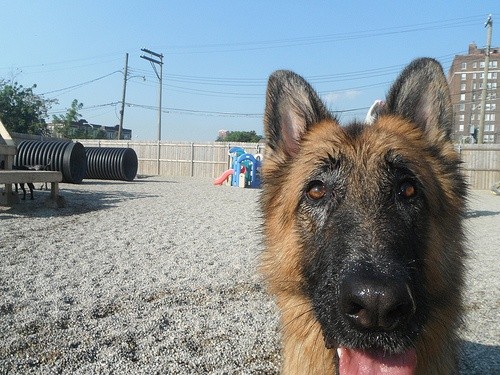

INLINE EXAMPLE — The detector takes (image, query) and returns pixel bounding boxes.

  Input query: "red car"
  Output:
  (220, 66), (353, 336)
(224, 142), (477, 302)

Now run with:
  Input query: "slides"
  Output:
(212, 168), (233, 185)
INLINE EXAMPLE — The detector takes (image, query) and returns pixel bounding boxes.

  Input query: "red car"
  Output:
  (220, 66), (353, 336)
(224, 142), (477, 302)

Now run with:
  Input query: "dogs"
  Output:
(257, 56), (467, 375)
